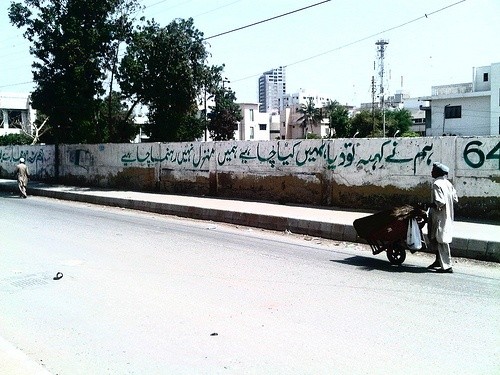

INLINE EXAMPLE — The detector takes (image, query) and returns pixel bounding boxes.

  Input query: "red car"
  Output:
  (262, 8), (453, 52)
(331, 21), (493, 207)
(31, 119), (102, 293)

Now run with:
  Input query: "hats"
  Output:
(19, 158), (25, 162)
(433, 161), (450, 173)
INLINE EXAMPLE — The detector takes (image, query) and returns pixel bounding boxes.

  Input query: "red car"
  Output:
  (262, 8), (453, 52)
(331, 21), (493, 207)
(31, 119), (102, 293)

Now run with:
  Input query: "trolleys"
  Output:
(354, 199), (429, 264)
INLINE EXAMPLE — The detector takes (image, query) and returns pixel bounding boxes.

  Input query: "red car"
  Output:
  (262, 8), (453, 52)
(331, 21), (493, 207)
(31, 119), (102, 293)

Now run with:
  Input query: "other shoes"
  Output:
(427, 261), (441, 269)
(436, 267), (453, 273)
(19, 195), (27, 198)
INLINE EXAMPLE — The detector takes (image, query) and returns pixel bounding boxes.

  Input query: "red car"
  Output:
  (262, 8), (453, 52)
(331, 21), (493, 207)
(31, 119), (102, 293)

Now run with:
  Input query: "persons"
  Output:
(421, 162), (458, 273)
(14, 158), (28, 199)
